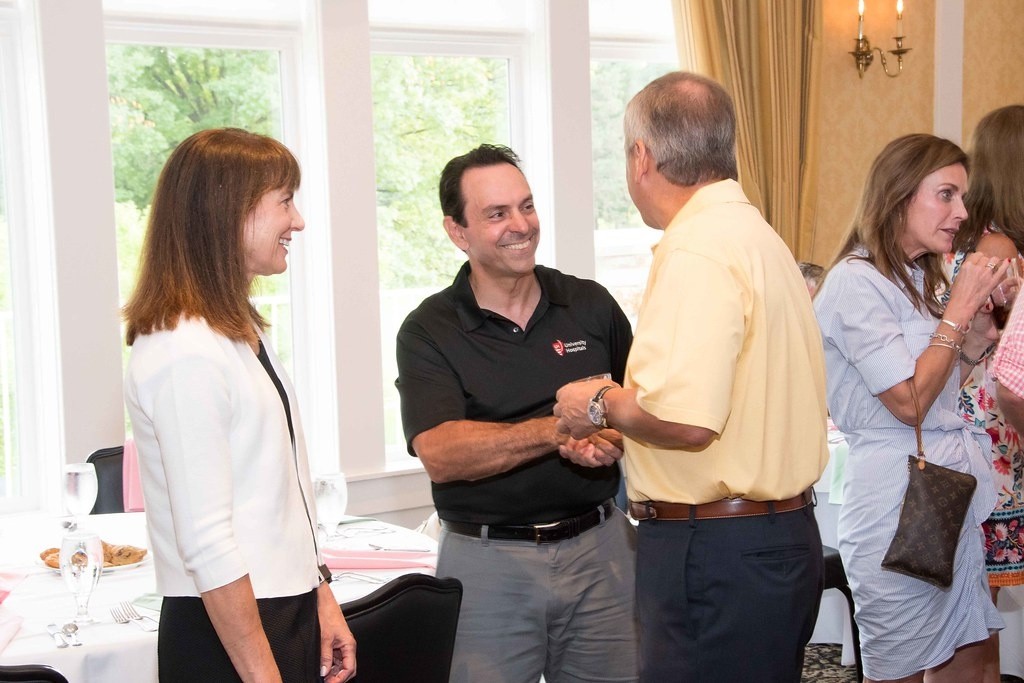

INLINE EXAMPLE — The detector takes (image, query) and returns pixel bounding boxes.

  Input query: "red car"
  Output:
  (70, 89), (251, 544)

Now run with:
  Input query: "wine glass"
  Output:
(61, 533), (104, 626)
(311, 471), (351, 558)
(60, 462), (99, 541)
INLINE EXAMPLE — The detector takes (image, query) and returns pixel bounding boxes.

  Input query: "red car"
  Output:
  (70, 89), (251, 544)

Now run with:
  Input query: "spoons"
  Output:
(64, 620), (83, 645)
(367, 543), (429, 551)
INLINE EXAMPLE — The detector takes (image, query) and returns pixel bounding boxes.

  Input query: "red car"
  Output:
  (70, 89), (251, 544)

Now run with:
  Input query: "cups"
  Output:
(989, 256), (1020, 308)
(567, 372), (613, 382)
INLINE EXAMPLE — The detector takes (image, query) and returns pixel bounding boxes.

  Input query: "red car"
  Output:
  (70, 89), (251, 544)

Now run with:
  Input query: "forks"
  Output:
(109, 599), (160, 633)
(336, 526), (396, 538)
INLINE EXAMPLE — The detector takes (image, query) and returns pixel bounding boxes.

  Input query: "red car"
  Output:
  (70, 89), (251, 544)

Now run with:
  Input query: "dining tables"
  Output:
(0, 511), (439, 683)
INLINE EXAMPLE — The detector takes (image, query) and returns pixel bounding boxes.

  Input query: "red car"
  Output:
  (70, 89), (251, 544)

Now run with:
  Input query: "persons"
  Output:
(396, 145), (638, 682)
(799, 104), (1024, 682)
(552, 71), (831, 683)
(125, 127), (358, 683)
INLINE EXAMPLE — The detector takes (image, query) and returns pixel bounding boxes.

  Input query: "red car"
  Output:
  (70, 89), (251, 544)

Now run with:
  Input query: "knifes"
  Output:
(46, 624), (67, 649)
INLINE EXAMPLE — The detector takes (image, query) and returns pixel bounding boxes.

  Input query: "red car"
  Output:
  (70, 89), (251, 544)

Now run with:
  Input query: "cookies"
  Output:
(39, 539), (147, 570)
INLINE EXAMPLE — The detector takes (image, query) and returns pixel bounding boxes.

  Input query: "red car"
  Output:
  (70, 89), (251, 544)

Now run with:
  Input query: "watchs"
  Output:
(588, 384), (614, 428)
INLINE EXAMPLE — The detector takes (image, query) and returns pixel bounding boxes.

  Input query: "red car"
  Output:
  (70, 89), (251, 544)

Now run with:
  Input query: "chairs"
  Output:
(339, 573), (464, 683)
(821, 543), (863, 683)
(85, 446), (124, 515)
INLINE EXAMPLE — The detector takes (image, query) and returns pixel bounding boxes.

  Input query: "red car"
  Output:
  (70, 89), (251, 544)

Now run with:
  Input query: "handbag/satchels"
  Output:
(880, 374), (977, 590)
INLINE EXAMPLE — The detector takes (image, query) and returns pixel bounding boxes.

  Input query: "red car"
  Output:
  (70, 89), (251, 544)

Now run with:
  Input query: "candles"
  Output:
(856, 0), (865, 39)
(893, 0), (905, 37)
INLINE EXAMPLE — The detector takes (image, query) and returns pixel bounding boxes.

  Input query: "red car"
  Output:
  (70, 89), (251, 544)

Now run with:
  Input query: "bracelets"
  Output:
(927, 316), (971, 361)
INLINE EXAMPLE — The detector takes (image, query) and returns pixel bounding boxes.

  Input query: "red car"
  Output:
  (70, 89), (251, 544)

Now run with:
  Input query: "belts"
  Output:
(629, 486), (814, 521)
(438, 497), (616, 547)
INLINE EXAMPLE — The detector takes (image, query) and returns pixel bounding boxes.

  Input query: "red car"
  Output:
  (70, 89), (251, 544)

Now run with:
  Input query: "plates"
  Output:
(35, 543), (153, 573)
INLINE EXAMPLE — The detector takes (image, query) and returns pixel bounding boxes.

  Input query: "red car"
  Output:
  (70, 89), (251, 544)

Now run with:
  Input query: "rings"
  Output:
(985, 263), (998, 272)
